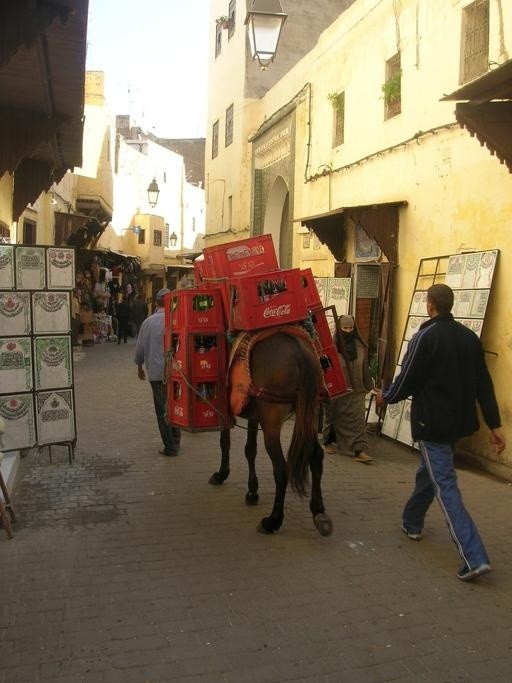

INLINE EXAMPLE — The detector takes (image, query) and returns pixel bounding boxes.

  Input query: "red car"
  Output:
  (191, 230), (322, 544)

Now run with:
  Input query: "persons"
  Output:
(322, 314), (376, 462)
(115, 296), (130, 344)
(134, 289), (181, 456)
(132, 294), (148, 335)
(374, 284), (506, 581)
(72, 289), (82, 346)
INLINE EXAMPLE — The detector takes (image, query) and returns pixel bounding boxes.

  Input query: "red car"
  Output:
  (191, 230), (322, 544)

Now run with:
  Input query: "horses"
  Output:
(208, 331), (334, 537)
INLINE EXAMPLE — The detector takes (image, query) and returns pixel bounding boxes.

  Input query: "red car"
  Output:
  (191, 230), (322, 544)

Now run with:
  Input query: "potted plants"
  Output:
(216, 16), (230, 29)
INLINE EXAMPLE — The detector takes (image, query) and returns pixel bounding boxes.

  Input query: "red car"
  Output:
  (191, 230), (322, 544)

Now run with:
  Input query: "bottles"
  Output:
(197, 383), (214, 400)
(194, 334), (217, 352)
(321, 357), (332, 370)
(301, 277), (308, 288)
(193, 295), (214, 311)
(257, 279), (287, 303)
(172, 335), (180, 353)
(173, 381), (181, 400)
(171, 296), (177, 312)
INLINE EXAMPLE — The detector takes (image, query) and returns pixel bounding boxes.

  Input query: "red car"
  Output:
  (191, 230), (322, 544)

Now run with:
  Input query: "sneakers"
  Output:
(456, 564), (491, 581)
(401, 526), (423, 539)
(325, 446), (335, 454)
(355, 452), (375, 463)
(160, 447), (174, 455)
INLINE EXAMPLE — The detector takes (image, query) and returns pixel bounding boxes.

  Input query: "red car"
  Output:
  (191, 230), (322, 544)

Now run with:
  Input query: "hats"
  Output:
(157, 288), (171, 300)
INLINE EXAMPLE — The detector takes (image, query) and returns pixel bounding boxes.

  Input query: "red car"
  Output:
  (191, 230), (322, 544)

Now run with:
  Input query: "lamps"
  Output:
(244, 0), (288, 71)
(170, 232), (177, 246)
(147, 177), (160, 208)
